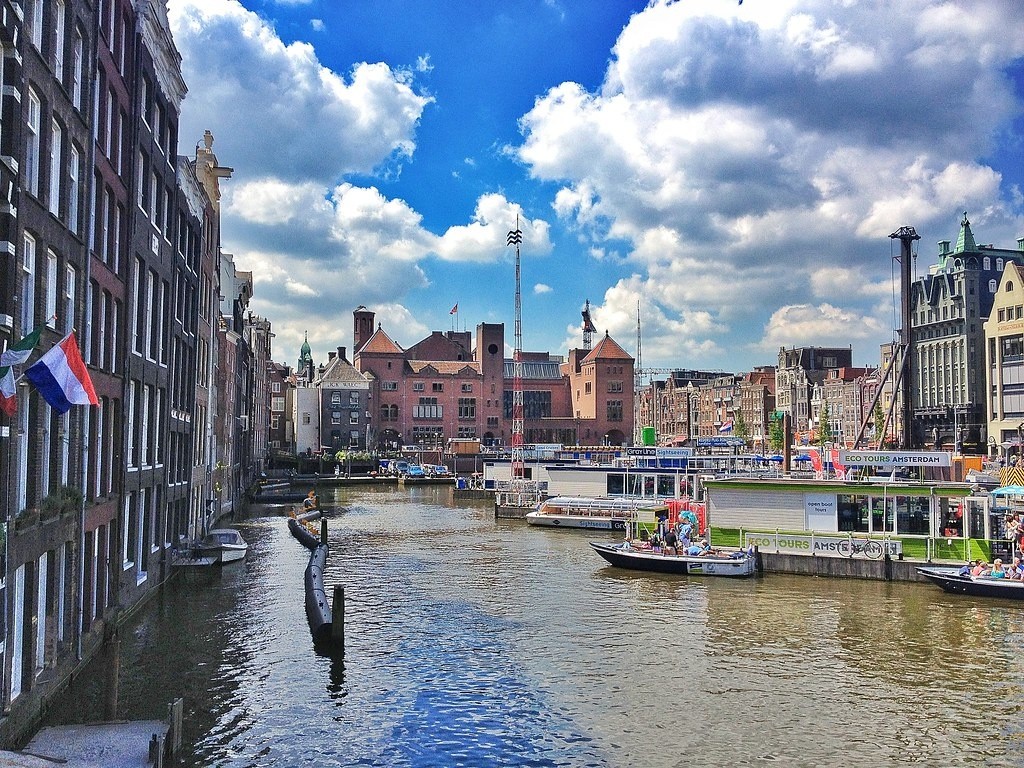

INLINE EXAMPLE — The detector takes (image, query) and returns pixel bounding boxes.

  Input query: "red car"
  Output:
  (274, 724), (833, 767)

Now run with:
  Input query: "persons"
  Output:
(359, 435), (1024, 578)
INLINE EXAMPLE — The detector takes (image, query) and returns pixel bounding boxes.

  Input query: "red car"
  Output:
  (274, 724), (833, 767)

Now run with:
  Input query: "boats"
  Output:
(525, 495), (660, 529)
(915, 566), (1024, 599)
(587, 538), (755, 577)
(194, 528), (247, 564)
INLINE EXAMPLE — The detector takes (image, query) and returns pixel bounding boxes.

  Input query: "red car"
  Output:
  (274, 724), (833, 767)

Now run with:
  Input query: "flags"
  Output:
(0, 320), (46, 421)
(718, 419), (732, 431)
(22, 331), (103, 418)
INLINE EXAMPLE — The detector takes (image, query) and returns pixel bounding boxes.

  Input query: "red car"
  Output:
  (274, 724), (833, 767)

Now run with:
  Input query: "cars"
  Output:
(370, 457), (455, 480)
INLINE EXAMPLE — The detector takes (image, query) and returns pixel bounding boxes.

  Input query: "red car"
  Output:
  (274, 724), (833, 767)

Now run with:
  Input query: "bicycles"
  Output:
(836, 529), (883, 559)
